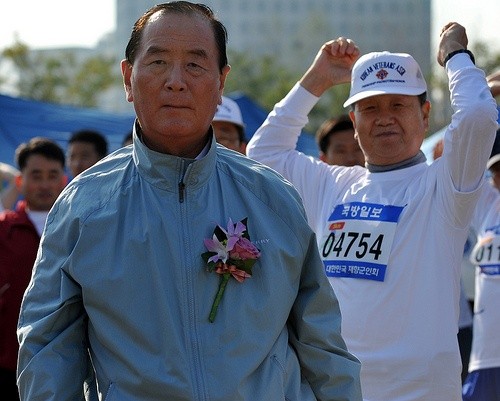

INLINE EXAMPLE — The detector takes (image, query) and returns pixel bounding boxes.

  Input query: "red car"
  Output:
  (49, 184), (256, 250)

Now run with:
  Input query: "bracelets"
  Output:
(443, 49), (475, 65)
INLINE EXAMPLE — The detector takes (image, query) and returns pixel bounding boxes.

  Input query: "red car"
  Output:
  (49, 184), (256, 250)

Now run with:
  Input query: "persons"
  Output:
(244, 22), (497, 401)
(0, 71), (499, 401)
(16, 2), (364, 401)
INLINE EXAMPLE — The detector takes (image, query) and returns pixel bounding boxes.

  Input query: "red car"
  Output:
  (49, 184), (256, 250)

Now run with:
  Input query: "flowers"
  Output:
(200, 217), (262, 324)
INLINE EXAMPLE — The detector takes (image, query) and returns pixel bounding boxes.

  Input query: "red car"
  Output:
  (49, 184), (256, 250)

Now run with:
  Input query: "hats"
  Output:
(343, 51), (428, 108)
(212, 94), (248, 132)
